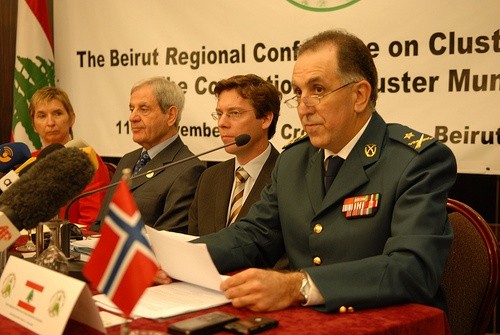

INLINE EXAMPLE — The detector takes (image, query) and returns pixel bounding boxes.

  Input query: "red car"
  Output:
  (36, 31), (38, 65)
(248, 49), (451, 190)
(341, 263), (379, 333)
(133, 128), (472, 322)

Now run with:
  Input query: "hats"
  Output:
(0, 142), (31, 174)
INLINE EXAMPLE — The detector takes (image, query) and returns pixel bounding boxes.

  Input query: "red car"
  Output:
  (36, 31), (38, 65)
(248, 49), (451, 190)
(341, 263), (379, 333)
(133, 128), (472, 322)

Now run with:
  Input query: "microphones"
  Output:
(0, 141), (93, 254)
(60, 134), (251, 258)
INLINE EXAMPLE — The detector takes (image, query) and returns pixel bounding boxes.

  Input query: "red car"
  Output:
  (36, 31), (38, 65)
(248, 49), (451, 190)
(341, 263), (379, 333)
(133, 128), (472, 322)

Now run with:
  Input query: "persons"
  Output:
(89, 76), (206, 241)
(187, 72), (284, 240)
(20, 86), (113, 237)
(149, 29), (458, 335)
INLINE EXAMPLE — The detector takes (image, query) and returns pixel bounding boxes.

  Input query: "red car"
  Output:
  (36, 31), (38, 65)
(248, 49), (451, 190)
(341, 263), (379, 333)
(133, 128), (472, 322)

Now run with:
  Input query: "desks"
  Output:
(0, 229), (451, 335)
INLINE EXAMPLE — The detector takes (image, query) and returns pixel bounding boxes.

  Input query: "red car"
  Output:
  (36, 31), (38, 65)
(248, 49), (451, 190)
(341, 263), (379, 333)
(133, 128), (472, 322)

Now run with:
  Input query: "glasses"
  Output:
(211, 109), (262, 121)
(285, 80), (361, 109)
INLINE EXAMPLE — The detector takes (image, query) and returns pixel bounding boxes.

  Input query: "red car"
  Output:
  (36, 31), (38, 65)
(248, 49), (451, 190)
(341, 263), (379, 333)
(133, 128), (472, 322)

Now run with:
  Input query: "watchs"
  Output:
(300, 271), (312, 302)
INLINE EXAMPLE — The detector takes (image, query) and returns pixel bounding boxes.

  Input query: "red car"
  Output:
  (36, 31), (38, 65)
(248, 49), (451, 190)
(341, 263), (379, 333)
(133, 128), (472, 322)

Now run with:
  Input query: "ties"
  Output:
(131, 150), (150, 173)
(323, 157), (344, 199)
(226, 168), (250, 229)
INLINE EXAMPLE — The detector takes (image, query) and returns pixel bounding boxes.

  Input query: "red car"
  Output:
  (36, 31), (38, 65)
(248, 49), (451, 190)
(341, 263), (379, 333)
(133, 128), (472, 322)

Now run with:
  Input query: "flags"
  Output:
(83, 181), (162, 319)
(8, 0), (56, 160)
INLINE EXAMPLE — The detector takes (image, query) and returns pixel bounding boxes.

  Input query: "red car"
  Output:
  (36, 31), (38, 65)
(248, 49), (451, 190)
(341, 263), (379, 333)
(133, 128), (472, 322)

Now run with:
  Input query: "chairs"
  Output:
(440, 198), (500, 335)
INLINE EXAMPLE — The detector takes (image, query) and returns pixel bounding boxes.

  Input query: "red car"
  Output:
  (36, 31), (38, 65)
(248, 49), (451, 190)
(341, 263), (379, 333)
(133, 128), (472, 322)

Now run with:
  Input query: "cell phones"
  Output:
(167, 311), (238, 335)
(224, 316), (280, 335)
